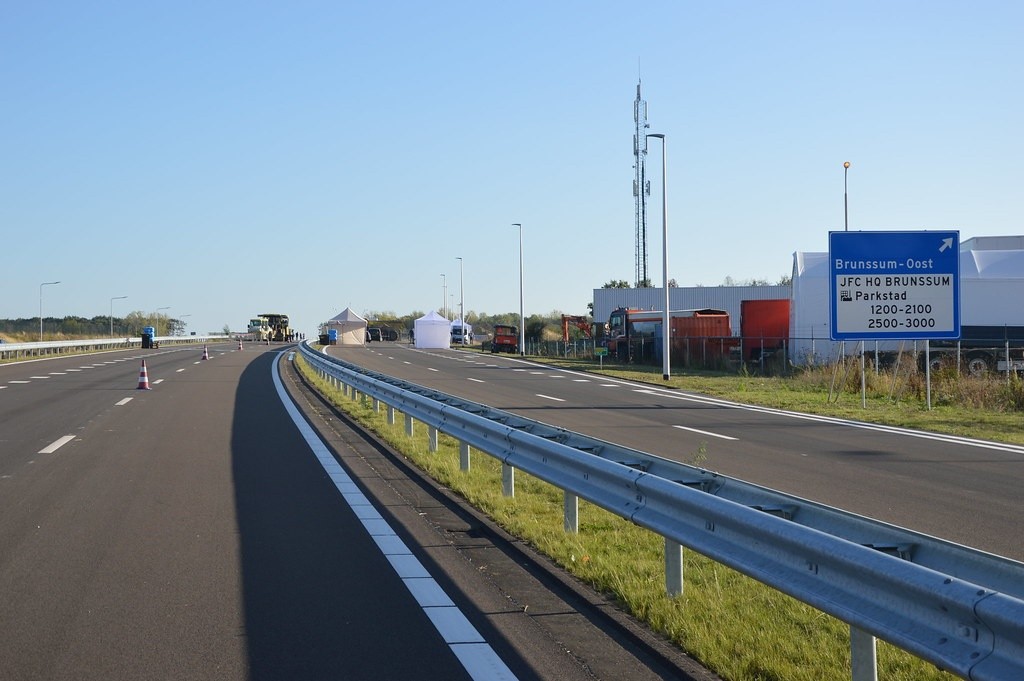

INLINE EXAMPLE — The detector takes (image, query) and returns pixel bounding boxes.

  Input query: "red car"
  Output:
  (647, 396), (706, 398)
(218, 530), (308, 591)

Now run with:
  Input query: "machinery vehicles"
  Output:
(558, 314), (611, 357)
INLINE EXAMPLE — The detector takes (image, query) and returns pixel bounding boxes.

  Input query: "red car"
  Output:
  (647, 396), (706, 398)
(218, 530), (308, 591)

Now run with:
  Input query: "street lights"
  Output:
(455, 256), (464, 348)
(110, 296), (128, 338)
(843, 161), (850, 230)
(156, 307), (170, 337)
(177, 314), (191, 335)
(646, 134), (671, 381)
(439, 273), (454, 323)
(511, 223), (525, 356)
(39, 281), (60, 341)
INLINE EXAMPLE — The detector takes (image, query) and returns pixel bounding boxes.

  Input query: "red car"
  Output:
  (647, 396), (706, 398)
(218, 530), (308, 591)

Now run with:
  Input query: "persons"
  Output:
(408, 330), (415, 344)
(300, 333), (306, 341)
(470, 331), (475, 345)
(295, 332), (299, 341)
(292, 329), (295, 341)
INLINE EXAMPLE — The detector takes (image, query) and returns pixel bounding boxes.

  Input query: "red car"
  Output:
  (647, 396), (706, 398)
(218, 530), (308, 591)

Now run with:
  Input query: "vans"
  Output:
(368, 328), (382, 342)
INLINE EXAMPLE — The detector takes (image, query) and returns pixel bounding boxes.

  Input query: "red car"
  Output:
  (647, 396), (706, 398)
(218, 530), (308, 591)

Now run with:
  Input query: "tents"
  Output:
(788, 250), (924, 366)
(328, 307), (368, 345)
(449, 318), (472, 335)
(413, 310), (451, 349)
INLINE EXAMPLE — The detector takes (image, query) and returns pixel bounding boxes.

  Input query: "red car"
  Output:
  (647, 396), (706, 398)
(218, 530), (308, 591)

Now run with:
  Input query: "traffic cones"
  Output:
(200, 344), (208, 360)
(238, 339), (243, 351)
(136, 360), (151, 389)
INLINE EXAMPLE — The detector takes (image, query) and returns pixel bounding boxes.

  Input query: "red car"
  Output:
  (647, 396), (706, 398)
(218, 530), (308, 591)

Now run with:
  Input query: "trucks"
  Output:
(248, 313), (293, 342)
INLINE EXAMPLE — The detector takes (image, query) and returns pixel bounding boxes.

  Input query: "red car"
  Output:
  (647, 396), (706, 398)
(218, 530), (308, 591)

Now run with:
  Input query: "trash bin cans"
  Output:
(318, 334), (329, 345)
(140, 333), (150, 349)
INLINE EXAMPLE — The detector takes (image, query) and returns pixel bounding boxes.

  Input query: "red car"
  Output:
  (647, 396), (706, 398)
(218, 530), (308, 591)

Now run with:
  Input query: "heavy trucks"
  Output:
(606, 306), (731, 361)
(450, 323), (471, 344)
(481, 324), (518, 353)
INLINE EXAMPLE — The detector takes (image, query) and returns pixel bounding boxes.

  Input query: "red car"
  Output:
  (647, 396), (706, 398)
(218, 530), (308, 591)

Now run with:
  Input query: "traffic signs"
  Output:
(828, 230), (960, 342)
